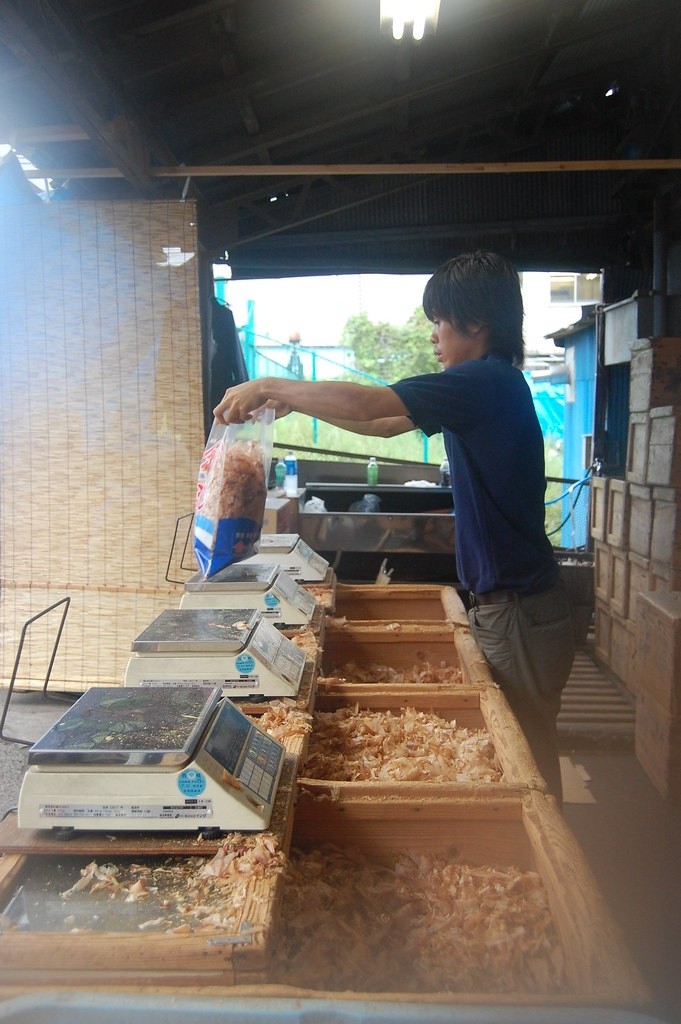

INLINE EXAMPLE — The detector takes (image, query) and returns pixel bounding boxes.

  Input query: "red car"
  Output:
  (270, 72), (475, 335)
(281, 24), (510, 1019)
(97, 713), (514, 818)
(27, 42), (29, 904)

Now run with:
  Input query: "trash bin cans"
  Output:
(555, 552), (596, 646)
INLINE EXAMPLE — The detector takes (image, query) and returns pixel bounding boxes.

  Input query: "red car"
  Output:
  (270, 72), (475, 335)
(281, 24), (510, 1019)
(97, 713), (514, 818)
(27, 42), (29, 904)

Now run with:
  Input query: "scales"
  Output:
(237, 532), (330, 584)
(179, 563), (318, 630)
(125, 609), (307, 704)
(18, 686), (287, 840)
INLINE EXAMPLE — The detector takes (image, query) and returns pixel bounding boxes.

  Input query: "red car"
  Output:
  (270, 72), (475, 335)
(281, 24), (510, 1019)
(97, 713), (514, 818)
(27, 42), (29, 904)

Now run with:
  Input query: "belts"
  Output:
(469, 571), (558, 608)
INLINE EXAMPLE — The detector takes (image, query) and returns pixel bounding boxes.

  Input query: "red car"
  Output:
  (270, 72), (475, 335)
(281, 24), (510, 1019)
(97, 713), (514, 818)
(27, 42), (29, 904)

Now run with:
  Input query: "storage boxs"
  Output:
(588, 335), (681, 805)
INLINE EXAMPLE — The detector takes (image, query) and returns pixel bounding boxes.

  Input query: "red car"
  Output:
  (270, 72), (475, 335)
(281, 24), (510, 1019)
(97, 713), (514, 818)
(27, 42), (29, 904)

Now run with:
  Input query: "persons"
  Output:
(213, 249), (577, 813)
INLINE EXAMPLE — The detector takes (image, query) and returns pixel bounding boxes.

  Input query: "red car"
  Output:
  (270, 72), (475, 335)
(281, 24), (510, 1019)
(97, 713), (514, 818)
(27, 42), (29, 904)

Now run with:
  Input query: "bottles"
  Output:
(367, 457), (378, 486)
(438, 458), (452, 488)
(274, 457), (287, 496)
(285, 450), (298, 498)
